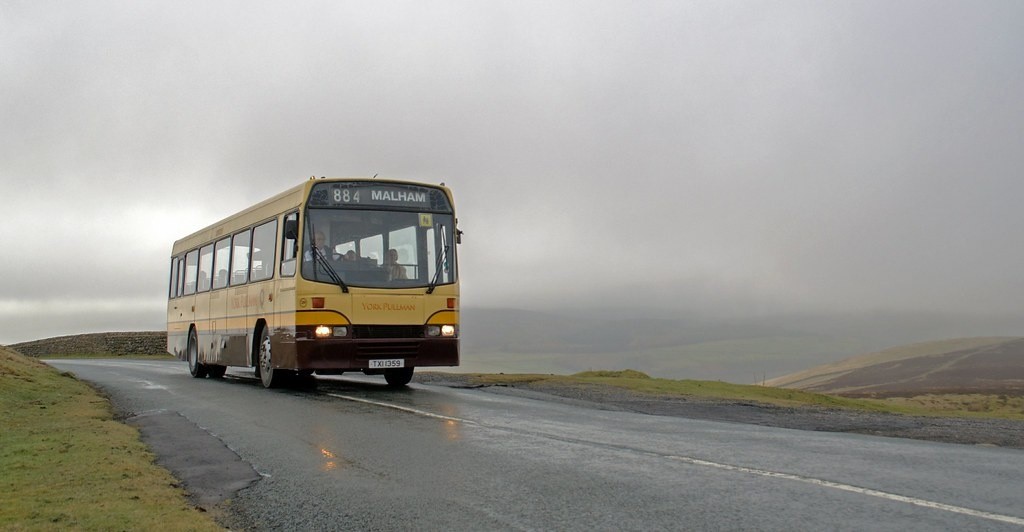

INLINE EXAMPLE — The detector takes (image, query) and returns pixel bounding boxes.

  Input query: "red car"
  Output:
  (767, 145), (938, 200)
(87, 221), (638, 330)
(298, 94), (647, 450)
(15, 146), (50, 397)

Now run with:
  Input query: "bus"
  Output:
(167, 174), (460, 388)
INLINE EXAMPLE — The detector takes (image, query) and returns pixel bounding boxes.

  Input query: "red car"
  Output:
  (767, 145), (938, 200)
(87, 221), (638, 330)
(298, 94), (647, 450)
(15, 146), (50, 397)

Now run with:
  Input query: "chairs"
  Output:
(186, 265), (263, 294)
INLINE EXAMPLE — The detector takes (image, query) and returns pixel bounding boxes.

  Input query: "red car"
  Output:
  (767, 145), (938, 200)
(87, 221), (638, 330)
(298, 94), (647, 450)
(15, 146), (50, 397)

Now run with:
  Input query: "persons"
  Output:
(342, 250), (357, 261)
(380, 249), (408, 280)
(200, 271), (209, 290)
(303, 232), (334, 261)
(219, 269), (228, 287)
